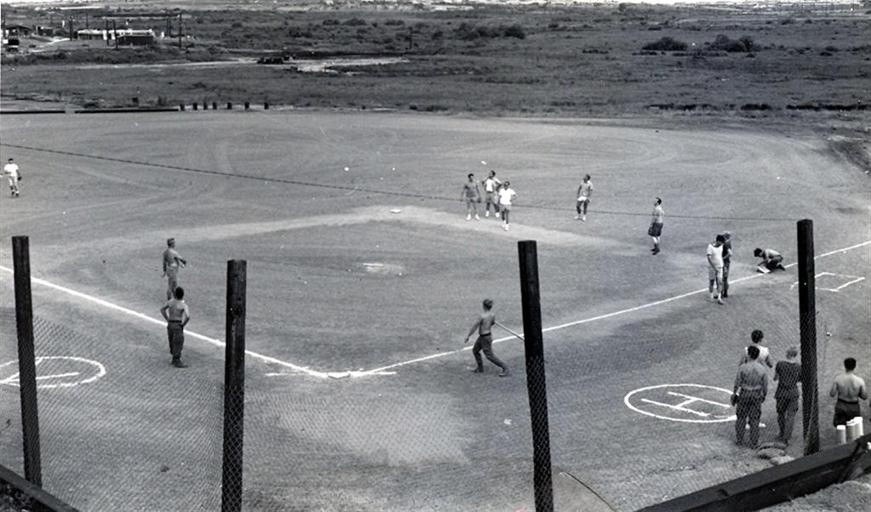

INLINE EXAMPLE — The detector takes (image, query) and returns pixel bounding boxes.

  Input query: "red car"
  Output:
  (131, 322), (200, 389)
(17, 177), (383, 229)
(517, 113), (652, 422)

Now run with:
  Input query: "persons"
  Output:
(161, 287), (190, 367)
(574, 174), (593, 221)
(464, 299), (509, 377)
(647, 196), (665, 254)
(461, 170), (518, 231)
(4, 158), (22, 197)
(738, 329), (774, 424)
(830, 358), (868, 427)
(706, 232), (785, 305)
(163, 238), (186, 300)
(733, 346), (770, 449)
(773, 344), (802, 444)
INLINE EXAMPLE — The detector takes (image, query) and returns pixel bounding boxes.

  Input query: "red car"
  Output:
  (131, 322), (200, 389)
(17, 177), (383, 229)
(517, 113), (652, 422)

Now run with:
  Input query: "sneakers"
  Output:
(575, 213), (581, 220)
(474, 367), (484, 372)
(500, 367), (511, 376)
(651, 245), (660, 255)
(467, 211), (509, 231)
(779, 264), (784, 271)
(710, 293), (727, 304)
(582, 215), (587, 221)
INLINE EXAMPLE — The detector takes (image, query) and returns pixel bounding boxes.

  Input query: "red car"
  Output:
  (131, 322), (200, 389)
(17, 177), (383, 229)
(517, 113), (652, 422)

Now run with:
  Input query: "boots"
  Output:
(176, 360), (188, 367)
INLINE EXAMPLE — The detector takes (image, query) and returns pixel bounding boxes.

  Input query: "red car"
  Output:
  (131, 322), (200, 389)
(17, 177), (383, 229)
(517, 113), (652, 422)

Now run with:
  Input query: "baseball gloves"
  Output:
(730, 394), (738, 407)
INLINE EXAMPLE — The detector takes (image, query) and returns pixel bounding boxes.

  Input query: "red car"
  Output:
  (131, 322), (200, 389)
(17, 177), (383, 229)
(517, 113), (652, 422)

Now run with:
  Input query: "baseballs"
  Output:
(480, 160), (488, 167)
(393, 167), (396, 172)
(343, 166), (351, 173)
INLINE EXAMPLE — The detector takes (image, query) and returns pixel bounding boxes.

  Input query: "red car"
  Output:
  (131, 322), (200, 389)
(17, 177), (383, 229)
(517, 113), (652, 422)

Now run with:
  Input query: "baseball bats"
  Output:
(494, 321), (527, 341)
